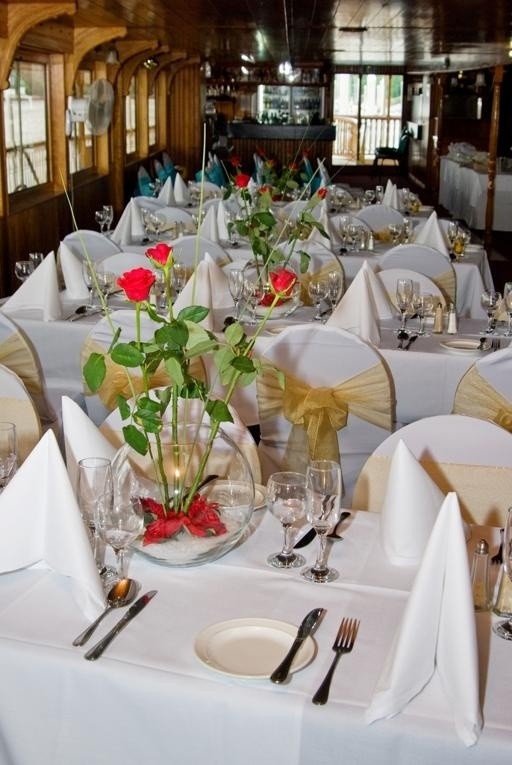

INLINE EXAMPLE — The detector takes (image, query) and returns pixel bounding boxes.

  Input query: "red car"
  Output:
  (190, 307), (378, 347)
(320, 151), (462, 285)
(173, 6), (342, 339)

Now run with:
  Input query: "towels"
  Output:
(386, 183), (400, 210)
(131, 197), (145, 236)
(174, 172), (190, 201)
(379, 439), (470, 566)
(197, 206), (218, 244)
(166, 260), (214, 331)
(325, 269), (381, 344)
(416, 211), (451, 261)
(217, 199), (231, 239)
(111, 202), (131, 245)
(1, 251), (62, 322)
(60, 396), (119, 504)
(307, 207), (341, 250)
(157, 176), (176, 205)
(205, 252), (236, 308)
(381, 179), (393, 203)
(62, 241), (90, 299)
(362, 260), (398, 318)
(363, 492), (483, 745)
(0, 428), (107, 621)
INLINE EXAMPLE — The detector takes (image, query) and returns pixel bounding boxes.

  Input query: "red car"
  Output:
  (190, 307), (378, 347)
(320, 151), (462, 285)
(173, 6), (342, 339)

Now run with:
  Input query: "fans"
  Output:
(66, 77), (114, 135)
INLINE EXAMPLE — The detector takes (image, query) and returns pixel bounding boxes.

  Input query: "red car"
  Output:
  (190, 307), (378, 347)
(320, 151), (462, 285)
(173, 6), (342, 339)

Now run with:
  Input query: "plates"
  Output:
(201, 481), (268, 510)
(439, 338), (490, 353)
(265, 326), (290, 335)
(194, 618), (316, 678)
(425, 313), (449, 319)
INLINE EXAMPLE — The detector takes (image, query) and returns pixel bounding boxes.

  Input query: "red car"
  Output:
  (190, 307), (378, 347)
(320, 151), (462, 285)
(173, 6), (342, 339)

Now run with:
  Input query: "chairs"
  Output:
(97, 387), (258, 485)
(162, 151), (177, 183)
(329, 215), (374, 241)
(97, 252), (166, 286)
(1, 312), (52, 421)
(78, 310), (206, 422)
(374, 269), (446, 318)
(454, 347), (510, 430)
(135, 165), (157, 197)
(259, 324), (395, 514)
(378, 243), (457, 307)
(0, 365), (41, 472)
(63, 229), (123, 263)
(352, 412), (510, 526)
(154, 160), (167, 182)
(152, 207), (197, 235)
(272, 237), (344, 302)
(355, 203), (404, 236)
(168, 235), (233, 279)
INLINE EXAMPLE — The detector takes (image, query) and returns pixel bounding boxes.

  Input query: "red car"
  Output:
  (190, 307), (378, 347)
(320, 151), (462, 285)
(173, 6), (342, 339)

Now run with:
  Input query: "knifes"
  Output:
(293, 528), (317, 549)
(70, 310), (96, 321)
(270, 608), (322, 683)
(403, 336), (418, 351)
(84, 591), (158, 660)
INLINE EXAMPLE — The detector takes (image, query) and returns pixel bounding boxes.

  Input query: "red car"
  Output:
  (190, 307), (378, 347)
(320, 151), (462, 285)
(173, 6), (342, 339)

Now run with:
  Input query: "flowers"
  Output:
(58, 122), (327, 512)
(228, 157), (328, 290)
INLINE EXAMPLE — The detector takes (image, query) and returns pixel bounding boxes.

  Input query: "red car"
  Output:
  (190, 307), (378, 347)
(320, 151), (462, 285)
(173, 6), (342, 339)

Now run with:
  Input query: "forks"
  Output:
(328, 511), (351, 541)
(312, 618), (357, 705)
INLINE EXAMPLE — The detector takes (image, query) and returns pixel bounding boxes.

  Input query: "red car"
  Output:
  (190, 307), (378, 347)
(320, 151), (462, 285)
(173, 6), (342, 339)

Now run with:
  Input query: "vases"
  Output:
(104, 422), (256, 568)
(241, 260), (301, 318)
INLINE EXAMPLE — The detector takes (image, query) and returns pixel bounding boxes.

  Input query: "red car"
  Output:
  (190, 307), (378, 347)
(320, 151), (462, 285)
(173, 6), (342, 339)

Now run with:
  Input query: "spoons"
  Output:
(71, 578), (137, 646)
(64, 305), (86, 320)
(397, 332), (409, 350)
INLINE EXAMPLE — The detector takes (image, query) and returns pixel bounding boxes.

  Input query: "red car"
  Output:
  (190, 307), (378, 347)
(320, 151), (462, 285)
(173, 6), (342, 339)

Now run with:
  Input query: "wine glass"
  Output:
(103, 205), (113, 232)
(140, 208), (150, 244)
(328, 271), (342, 311)
(189, 181), (203, 205)
(243, 282), (264, 325)
(389, 223), (402, 247)
(412, 293), (433, 337)
(348, 226), (361, 253)
(504, 283), (512, 335)
(15, 262), (34, 282)
(302, 461), (342, 583)
(394, 279), (413, 334)
(366, 190), (374, 205)
(77, 457), (115, 534)
(479, 291), (501, 335)
(83, 260), (97, 308)
(454, 236), (464, 262)
(403, 218), (412, 243)
(154, 270), (173, 310)
(95, 211), (107, 234)
(266, 472), (307, 567)
(96, 272), (115, 313)
(225, 210), (236, 246)
(0, 422), (16, 491)
(327, 183), (350, 212)
(338, 217), (350, 254)
(173, 264), (186, 297)
(309, 280), (329, 322)
(403, 188), (418, 214)
(492, 507), (512, 640)
(376, 186), (384, 205)
(148, 178), (163, 197)
(304, 183), (311, 199)
(149, 213), (165, 244)
(30, 254), (43, 267)
(94, 495), (143, 592)
(448, 222), (458, 259)
(229, 269), (244, 322)
